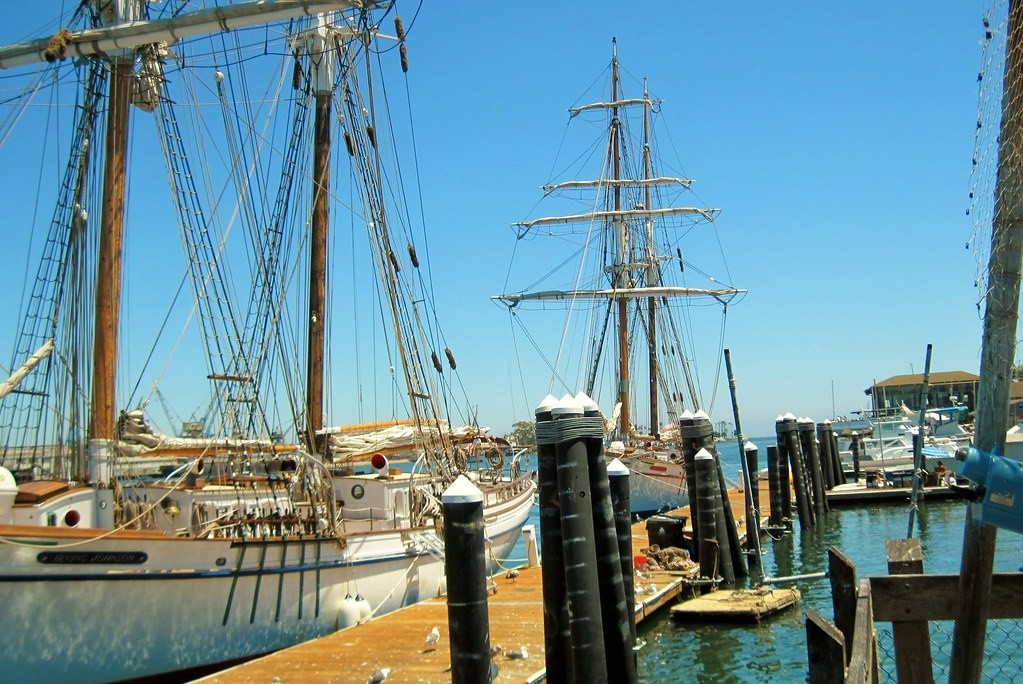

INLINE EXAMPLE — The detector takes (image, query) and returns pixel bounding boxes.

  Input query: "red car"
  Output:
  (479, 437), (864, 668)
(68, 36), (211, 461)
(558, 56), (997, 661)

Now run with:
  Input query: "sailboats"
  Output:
(489, 33), (750, 526)
(0, 0), (537, 681)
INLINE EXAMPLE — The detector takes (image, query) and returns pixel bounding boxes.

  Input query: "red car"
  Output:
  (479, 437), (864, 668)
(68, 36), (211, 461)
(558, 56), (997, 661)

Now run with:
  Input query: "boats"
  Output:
(837, 407), (974, 477)
